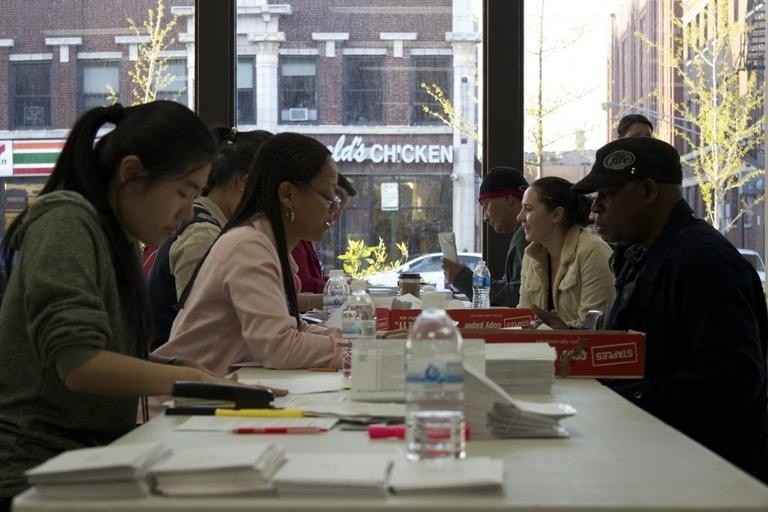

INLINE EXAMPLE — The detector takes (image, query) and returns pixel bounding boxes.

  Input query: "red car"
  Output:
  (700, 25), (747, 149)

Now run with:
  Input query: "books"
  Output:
(466, 338), (578, 441)
(23, 436), (506, 502)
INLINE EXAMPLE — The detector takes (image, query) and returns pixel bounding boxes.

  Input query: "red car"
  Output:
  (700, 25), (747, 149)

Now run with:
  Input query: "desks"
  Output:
(12, 291), (768, 511)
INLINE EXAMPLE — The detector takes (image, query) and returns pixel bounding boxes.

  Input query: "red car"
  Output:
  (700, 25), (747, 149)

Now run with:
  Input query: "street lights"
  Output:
(600, 100), (724, 231)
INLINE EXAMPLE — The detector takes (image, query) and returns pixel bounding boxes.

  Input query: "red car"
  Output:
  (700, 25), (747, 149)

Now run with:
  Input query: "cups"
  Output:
(398, 272), (421, 298)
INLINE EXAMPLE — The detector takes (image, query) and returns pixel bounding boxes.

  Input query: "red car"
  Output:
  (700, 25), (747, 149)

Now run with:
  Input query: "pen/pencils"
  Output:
(232, 424), (328, 434)
(214, 408), (304, 417)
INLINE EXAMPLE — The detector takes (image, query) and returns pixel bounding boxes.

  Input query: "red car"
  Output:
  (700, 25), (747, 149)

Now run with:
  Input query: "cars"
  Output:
(362, 250), (483, 301)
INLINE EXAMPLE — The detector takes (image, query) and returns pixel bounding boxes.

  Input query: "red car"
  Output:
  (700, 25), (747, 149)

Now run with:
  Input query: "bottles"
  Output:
(405, 287), (466, 461)
(342, 279), (375, 396)
(320, 268), (350, 315)
(470, 260), (491, 308)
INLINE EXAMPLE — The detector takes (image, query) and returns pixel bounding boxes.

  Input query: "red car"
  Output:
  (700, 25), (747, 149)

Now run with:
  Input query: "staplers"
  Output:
(165, 381), (275, 415)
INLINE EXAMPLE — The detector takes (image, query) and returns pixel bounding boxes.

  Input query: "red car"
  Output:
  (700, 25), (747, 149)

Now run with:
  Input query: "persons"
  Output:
(132, 129), (408, 428)
(0, 97), (291, 512)
(293, 172), (358, 295)
(440, 167), (531, 307)
(616, 115), (655, 140)
(569, 136), (768, 485)
(515, 177), (619, 330)
(146, 123), (274, 352)
(292, 291), (324, 314)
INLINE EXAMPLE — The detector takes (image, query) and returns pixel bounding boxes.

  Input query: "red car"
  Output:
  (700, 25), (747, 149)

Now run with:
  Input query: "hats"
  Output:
(478, 167), (529, 202)
(568, 136), (682, 196)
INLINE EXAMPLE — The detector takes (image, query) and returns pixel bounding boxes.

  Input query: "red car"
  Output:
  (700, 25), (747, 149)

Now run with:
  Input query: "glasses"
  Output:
(304, 183), (340, 208)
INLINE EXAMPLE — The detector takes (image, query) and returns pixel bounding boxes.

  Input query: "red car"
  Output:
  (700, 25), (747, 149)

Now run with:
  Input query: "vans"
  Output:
(737, 245), (766, 296)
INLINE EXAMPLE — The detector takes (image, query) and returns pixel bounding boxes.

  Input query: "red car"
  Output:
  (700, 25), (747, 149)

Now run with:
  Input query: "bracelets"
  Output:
(305, 292), (312, 312)
